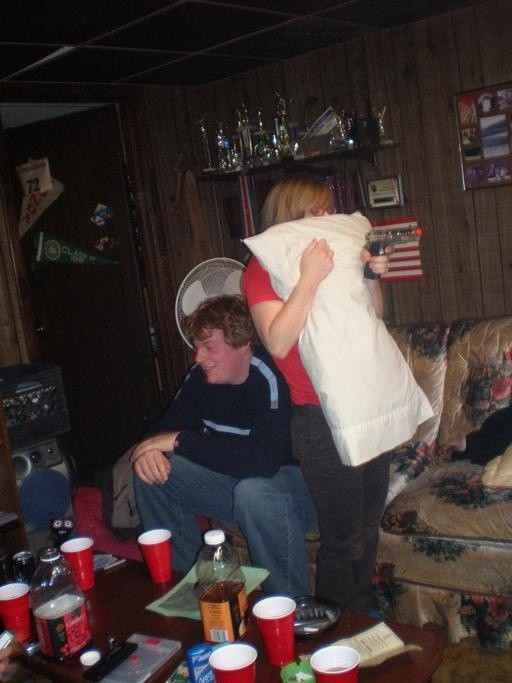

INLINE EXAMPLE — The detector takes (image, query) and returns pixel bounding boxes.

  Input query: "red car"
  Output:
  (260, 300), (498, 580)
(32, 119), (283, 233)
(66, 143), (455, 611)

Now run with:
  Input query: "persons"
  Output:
(130, 295), (320, 609)
(242, 172), (393, 619)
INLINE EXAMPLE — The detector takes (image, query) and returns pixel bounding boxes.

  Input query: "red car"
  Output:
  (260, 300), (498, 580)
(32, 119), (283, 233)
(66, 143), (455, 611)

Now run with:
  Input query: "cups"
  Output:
(209, 642), (261, 682)
(59, 537), (104, 593)
(278, 660), (313, 683)
(1, 582), (36, 646)
(137, 529), (173, 582)
(78, 650), (103, 672)
(307, 644), (360, 682)
(246, 595), (300, 669)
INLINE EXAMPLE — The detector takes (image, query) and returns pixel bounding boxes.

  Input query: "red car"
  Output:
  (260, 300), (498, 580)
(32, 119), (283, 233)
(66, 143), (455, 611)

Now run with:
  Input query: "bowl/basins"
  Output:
(292, 594), (346, 643)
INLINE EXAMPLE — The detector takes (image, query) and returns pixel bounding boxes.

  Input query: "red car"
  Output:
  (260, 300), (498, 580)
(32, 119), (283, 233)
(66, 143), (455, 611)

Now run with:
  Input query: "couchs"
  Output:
(208, 313), (512, 653)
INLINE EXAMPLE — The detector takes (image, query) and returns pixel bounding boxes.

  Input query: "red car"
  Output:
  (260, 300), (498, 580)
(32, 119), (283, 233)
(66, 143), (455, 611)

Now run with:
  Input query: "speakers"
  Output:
(0, 361), (73, 452)
(9, 436), (77, 550)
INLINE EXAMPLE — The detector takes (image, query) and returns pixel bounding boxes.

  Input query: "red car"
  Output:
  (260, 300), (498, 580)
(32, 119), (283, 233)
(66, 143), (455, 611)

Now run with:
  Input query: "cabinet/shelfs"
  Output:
(0, 410), (29, 556)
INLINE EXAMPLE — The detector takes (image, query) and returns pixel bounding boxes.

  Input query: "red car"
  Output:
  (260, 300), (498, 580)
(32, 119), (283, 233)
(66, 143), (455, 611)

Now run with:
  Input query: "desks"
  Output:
(1, 551), (448, 683)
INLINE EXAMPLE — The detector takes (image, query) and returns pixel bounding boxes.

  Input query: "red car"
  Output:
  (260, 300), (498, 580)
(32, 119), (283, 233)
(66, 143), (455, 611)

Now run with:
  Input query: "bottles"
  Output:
(31, 546), (99, 663)
(194, 528), (253, 644)
(194, 86), (394, 177)
(189, 646), (215, 682)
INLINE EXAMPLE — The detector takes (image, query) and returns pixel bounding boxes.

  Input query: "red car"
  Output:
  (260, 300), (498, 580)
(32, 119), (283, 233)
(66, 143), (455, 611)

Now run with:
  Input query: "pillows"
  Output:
(239, 211), (435, 469)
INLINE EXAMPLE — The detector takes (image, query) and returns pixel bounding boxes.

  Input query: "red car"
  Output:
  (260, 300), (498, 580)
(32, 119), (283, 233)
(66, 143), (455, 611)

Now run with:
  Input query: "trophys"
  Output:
(192, 90), (297, 171)
